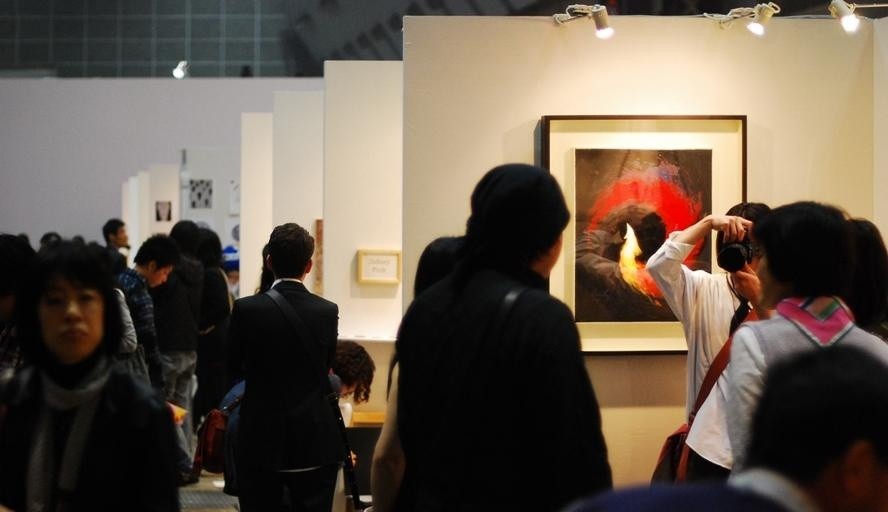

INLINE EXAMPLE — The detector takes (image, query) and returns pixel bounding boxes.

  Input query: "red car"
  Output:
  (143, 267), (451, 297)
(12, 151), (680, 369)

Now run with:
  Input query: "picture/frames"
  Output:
(537, 113), (749, 355)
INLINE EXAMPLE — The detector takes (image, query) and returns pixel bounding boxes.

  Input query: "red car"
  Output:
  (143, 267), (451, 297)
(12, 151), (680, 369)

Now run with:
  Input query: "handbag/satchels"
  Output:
(192, 405), (233, 478)
(651, 422), (693, 491)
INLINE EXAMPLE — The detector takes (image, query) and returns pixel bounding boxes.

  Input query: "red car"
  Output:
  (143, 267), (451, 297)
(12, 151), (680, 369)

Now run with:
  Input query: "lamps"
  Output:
(704, 1), (781, 38)
(832, 1), (887, 35)
(551, 5), (613, 38)
(171, 60), (190, 78)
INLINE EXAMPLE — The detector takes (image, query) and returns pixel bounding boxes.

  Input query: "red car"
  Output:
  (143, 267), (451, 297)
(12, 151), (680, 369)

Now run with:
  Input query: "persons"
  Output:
(644, 202), (783, 487)
(395, 162), (614, 510)
(371, 235), (464, 511)
(562, 343), (888, 510)
(0, 222), (233, 512)
(840, 219), (886, 338)
(223, 223), (347, 510)
(684, 201), (888, 481)
(215, 340), (375, 498)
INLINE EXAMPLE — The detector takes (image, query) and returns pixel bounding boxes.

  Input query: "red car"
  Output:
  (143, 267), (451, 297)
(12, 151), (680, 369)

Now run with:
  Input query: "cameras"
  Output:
(716, 227), (753, 272)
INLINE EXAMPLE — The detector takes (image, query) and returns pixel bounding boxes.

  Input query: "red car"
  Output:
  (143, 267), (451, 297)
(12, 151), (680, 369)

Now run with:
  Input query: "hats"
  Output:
(467, 162), (573, 255)
